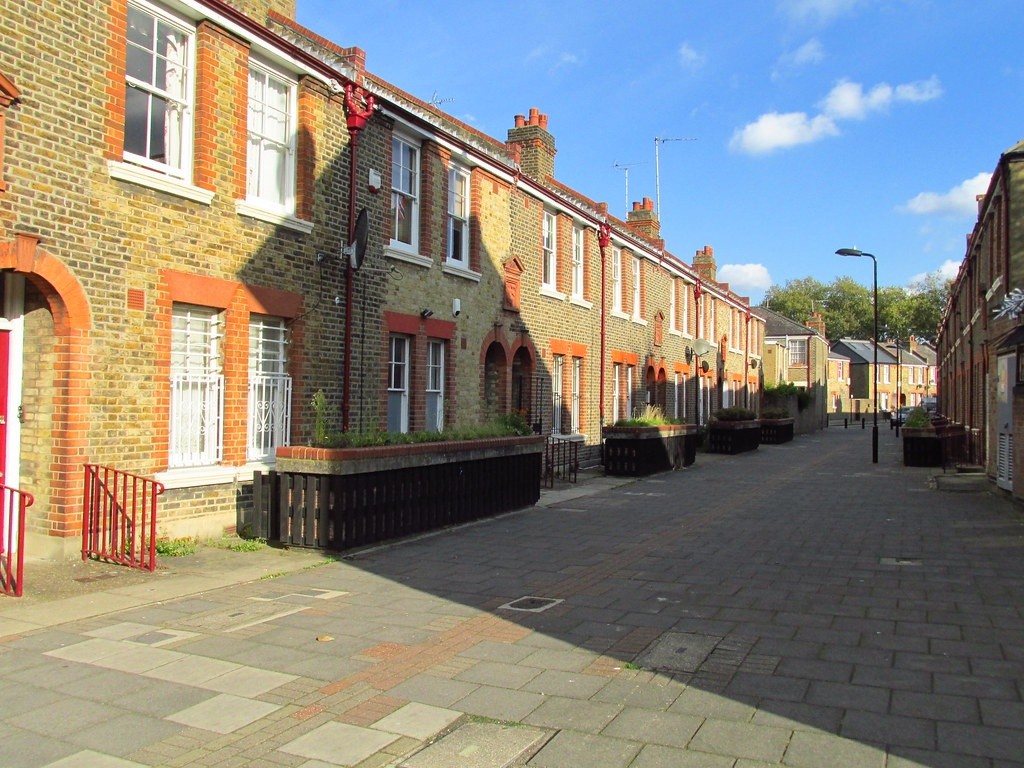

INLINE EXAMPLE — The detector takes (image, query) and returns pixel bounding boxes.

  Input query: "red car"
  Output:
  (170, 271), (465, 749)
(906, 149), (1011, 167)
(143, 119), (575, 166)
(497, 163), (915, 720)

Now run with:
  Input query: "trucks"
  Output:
(921, 397), (936, 414)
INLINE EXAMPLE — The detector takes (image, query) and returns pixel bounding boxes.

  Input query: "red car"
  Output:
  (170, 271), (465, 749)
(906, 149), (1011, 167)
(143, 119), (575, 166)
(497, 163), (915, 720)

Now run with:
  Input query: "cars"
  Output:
(890, 406), (915, 427)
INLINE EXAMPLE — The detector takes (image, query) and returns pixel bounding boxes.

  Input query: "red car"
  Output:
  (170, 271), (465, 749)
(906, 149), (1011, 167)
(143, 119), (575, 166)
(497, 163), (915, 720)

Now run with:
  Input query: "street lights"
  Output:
(834, 248), (878, 464)
(885, 345), (902, 427)
